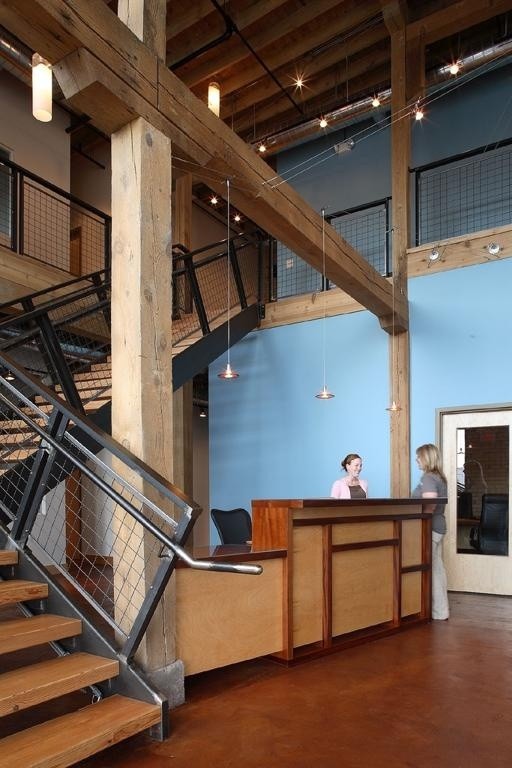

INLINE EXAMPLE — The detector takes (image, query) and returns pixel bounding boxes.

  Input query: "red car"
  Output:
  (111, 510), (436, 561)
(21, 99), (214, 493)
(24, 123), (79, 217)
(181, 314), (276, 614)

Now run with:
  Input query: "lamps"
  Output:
(199, 407), (208, 417)
(216, 175), (240, 380)
(4, 367), (15, 381)
(314, 207), (337, 402)
(30, 50), (55, 124)
(206, 80), (222, 121)
(385, 225), (403, 412)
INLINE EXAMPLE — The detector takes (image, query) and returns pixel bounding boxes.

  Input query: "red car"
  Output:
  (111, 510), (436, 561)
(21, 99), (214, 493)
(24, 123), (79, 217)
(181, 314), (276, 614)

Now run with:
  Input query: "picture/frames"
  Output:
(36, 492), (47, 516)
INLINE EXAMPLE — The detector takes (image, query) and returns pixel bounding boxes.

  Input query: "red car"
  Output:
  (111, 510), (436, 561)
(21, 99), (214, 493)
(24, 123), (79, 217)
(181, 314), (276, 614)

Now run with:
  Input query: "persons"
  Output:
(463, 461), (487, 553)
(411, 443), (450, 621)
(331, 453), (369, 499)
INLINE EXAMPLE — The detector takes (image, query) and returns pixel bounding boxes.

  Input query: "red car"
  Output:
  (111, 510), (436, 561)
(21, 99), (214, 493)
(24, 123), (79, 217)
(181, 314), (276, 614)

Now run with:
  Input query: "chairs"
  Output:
(469, 491), (509, 555)
(210, 505), (253, 544)
